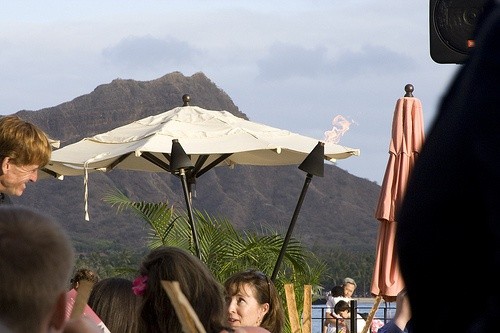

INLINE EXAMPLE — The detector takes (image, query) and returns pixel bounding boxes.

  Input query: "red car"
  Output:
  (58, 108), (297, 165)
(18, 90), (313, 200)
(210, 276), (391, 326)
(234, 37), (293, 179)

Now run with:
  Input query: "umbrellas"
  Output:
(367, 84), (426, 300)
(36, 93), (361, 256)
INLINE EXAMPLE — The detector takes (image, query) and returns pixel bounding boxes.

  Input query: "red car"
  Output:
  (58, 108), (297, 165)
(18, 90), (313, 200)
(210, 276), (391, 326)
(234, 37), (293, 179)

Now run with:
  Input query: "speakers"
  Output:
(429, 0), (489, 64)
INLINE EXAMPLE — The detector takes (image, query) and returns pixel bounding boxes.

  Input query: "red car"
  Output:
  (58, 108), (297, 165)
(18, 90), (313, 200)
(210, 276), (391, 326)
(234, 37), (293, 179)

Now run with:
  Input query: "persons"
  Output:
(134, 245), (226, 333)
(325, 277), (357, 333)
(323, 285), (354, 333)
(88, 278), (133, 333)
(334, 300), (369, 333)
(377, 288), (411, 333)
(1, 114), (52, 205)
(224, 270), (285, 333)
(0, 204), (77, 333)
